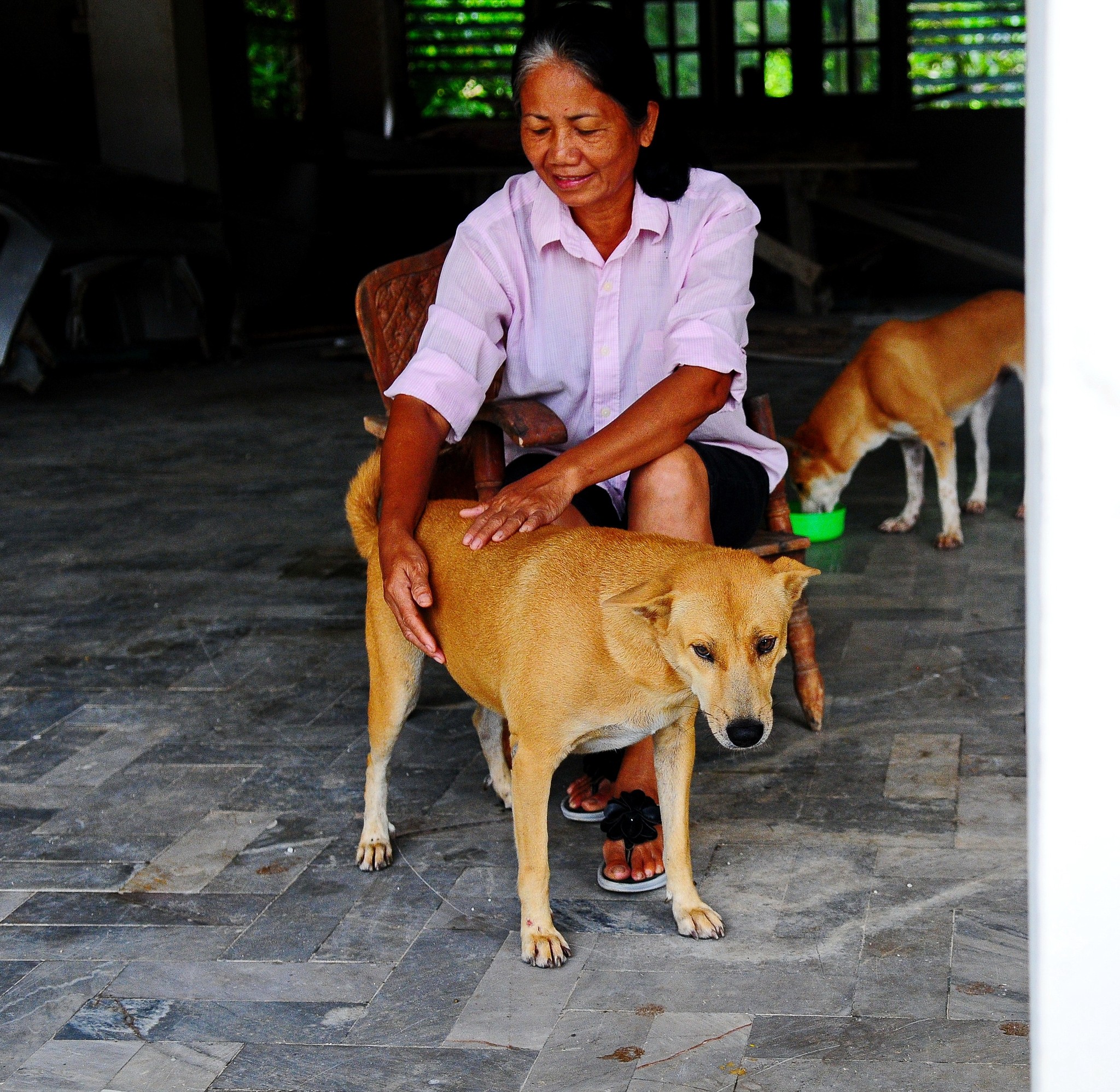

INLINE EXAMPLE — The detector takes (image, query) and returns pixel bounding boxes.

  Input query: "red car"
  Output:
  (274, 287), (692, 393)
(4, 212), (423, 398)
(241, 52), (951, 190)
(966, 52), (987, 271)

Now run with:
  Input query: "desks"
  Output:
(710, 157), (929, 355)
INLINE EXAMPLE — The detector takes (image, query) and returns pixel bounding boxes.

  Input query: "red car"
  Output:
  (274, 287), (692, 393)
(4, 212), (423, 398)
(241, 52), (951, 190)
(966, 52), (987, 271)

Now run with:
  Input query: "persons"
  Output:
(378, 1), (788, 892)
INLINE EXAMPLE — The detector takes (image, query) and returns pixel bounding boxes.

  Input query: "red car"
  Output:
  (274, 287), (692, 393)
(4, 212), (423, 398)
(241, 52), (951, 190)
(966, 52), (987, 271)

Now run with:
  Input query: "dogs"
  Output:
(789, 291), (1026, 552)
(337, 405), (821, 971)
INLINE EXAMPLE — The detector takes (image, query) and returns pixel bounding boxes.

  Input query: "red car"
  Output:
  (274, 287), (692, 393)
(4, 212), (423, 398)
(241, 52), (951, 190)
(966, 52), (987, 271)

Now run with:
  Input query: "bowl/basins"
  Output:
(787, 503), (847, 542)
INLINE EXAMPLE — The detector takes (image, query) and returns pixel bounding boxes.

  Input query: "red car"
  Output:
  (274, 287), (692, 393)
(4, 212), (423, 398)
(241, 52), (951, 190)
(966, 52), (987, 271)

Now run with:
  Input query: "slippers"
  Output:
(597, 788), (667, 893)
(558, 753), (622, 823)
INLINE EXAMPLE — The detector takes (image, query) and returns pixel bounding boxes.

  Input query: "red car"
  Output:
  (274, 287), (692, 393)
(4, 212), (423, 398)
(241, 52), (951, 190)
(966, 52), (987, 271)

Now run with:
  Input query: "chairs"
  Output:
(356, 239), (827, 783)
